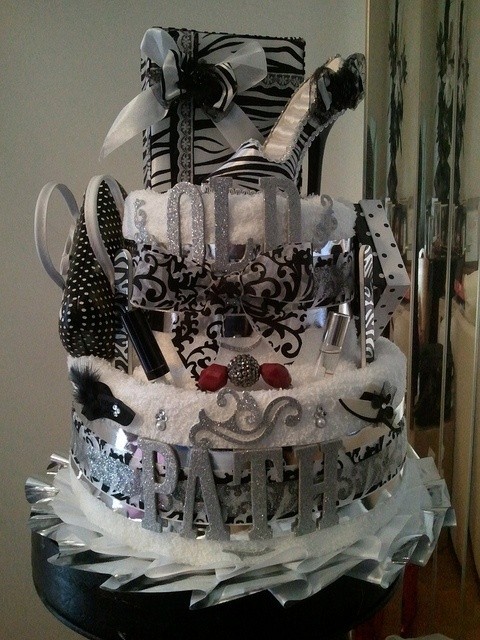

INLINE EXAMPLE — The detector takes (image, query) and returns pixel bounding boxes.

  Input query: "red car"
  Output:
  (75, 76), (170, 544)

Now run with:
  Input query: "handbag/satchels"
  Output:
(33, 175), (138, 374)
